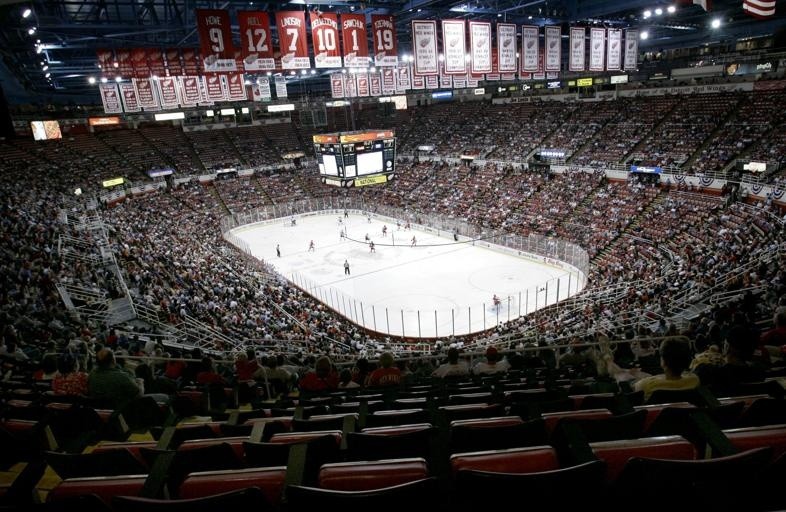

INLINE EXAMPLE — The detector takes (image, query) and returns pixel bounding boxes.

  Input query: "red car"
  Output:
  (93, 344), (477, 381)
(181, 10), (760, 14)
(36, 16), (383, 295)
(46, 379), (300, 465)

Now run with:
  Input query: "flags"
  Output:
(742, 0), (776, 19)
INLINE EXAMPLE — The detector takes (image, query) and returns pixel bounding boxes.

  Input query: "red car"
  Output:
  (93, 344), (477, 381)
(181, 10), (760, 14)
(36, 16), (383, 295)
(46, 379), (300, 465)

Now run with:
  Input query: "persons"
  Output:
(0, 78), (786, 405)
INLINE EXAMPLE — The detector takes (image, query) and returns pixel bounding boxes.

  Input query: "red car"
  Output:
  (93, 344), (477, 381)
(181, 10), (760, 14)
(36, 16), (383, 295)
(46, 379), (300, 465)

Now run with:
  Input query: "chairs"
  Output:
(0, 361), (785, 510)
